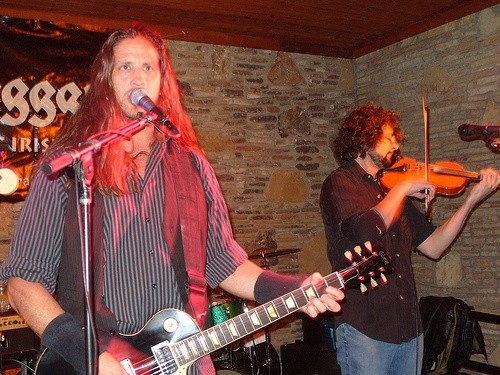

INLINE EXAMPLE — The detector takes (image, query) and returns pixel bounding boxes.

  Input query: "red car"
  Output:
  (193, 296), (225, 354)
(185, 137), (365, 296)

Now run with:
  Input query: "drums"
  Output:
(207, 298), (245, 326)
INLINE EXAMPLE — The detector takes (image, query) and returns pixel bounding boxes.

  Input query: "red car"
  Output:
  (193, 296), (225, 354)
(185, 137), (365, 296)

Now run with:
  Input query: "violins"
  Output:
(376, 156), (482, 196)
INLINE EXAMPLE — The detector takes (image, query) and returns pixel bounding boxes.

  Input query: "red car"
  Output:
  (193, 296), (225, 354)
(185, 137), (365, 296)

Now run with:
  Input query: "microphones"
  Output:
(458, 123), (500, 142)
(130, 88), (180, 134)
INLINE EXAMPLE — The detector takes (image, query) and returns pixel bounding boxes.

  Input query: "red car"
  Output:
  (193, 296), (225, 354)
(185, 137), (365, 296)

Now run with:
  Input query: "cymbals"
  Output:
(246, 248), (305, 260)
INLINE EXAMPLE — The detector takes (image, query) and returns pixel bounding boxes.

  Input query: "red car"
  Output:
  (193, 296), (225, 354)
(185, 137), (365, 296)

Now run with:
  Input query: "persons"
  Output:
(0, 21), (345, 375)
(320, 101), (500, 374)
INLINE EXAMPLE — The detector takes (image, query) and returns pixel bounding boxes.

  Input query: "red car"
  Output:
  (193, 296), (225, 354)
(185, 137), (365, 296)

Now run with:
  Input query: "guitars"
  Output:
(31, 240), (393, 375)
